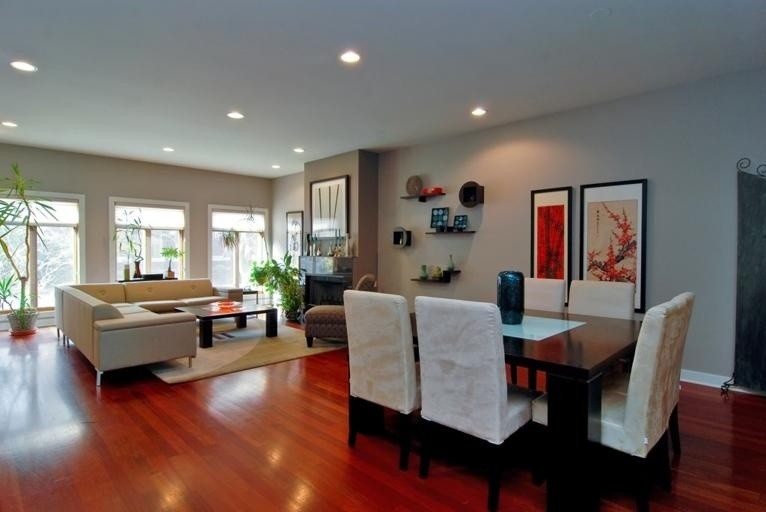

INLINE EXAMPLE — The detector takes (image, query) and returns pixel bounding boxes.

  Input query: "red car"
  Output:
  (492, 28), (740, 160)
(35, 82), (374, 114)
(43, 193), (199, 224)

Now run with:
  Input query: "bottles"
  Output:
(448, 254), (453, 271)
(124, 264), (129, 281)
(418, 264), (428, 279)
(309, 236), (343, 256)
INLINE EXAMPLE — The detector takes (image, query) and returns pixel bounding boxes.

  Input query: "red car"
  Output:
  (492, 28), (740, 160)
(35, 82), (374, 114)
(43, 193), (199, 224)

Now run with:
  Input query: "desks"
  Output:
(240, 287), (259, 319)
(113, 274), (179, 284)
(411, 308), (681, 509)
(304, 269), (352, 321)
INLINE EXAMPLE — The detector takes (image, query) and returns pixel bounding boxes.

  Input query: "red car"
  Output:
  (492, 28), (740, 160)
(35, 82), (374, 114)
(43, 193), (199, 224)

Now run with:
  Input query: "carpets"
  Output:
(140, 313), (348, 390)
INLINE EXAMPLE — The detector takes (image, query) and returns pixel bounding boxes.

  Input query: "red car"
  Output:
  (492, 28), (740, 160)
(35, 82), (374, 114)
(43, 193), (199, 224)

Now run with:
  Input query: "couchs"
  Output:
(47, 275), (197, 391)
(120, 278), (244, 315)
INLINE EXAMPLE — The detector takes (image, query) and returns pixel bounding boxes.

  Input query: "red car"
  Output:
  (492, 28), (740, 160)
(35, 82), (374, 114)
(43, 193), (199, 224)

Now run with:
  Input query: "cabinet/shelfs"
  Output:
(398, 190), (479, 286)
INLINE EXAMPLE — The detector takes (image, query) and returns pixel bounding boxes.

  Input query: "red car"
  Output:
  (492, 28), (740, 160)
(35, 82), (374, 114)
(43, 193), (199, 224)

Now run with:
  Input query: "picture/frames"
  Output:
(527, 184), (572, 308)
(308, 173), (351, 241)
(575, 176), (649, 313)
(285, 209), (304, 257)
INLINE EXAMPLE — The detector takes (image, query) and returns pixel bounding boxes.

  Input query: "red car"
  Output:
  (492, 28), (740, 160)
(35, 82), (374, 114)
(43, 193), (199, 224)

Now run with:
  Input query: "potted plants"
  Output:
(111, 204), (155, 278)
(118, 240), (130, 281)
(160, 244), (188, 279)
(246, 251), (304, 327)
(0, 160), (60, 339)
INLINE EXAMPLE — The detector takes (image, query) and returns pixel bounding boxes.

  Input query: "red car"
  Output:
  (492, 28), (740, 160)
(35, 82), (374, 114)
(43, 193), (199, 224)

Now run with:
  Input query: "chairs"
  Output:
(301, 272), (379, 349)
(531, 290), (699, 509)
(523, 277), (567, 312)
(567, 280), (636, 320)
(414, 295), (543, 510)
(342, 289), (422, 472)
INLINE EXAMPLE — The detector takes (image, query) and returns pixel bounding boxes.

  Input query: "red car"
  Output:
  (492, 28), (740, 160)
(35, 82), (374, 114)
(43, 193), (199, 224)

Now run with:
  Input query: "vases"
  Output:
(326, 244), (334, 256)
(418, 263), (428, 280)
(446, 254), (455, 272)
(315, 247), (321, 256)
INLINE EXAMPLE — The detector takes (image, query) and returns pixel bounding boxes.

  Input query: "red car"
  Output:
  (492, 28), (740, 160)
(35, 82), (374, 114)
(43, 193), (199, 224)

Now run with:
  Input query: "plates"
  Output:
(210, 302), (240, 308)
(405, 175), (422, 195)
(210, 307), (241, 314)
